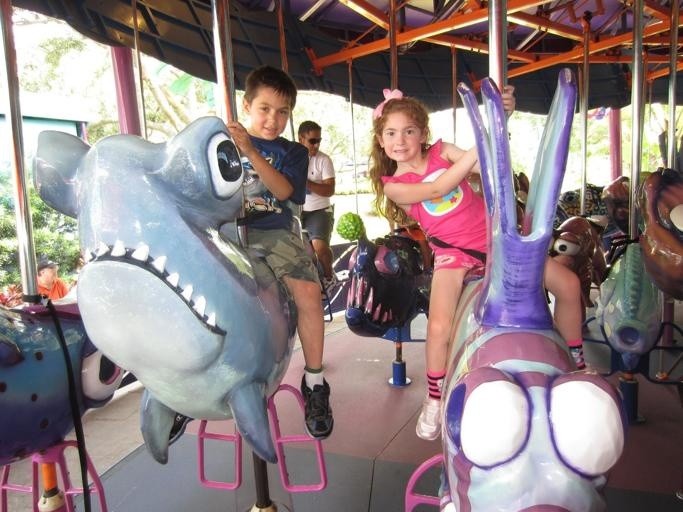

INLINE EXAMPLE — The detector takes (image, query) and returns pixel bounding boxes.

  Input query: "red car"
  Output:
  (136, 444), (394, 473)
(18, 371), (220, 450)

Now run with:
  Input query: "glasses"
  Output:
(308, 138), (320, 144)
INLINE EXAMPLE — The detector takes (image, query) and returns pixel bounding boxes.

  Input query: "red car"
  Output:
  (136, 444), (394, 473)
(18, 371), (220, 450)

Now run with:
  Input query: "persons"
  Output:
(36, 259), (67, 301)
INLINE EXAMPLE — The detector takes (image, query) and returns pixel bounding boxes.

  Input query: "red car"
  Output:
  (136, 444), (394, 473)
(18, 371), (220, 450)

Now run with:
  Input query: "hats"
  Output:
(37, 259), (60, 272)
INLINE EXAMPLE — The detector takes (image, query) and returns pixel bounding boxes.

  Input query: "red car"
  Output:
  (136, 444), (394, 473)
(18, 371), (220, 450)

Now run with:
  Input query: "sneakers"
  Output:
(321, 273), (337, 301)
(300, 374), (333, 441)
(415, 391), (441, 441)
(168, 412), (194, 446)
